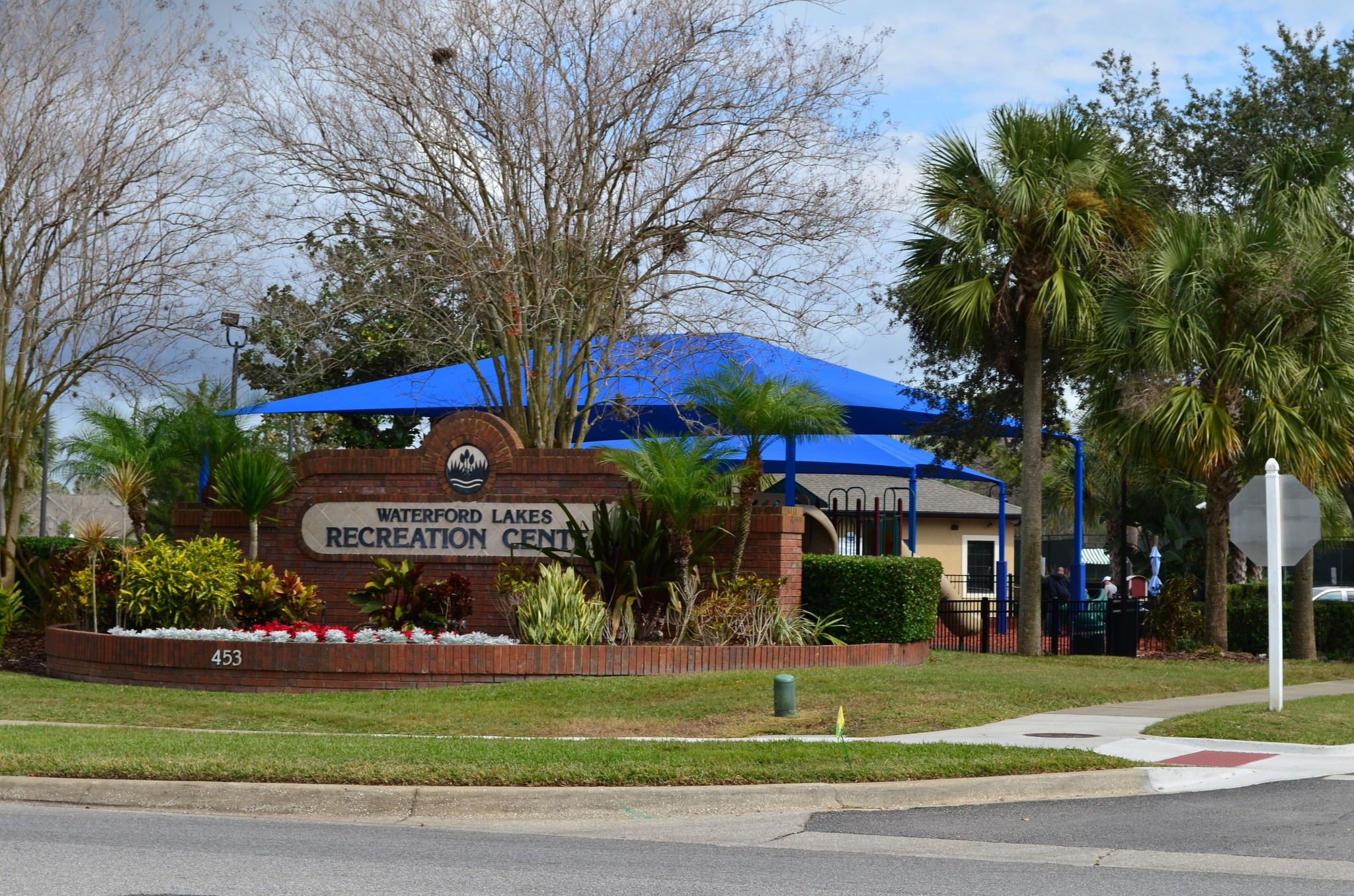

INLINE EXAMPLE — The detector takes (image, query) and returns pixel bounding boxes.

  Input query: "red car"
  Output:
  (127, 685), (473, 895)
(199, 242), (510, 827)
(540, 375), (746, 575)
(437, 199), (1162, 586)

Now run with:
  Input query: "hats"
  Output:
(1101, 576), (1111, 585)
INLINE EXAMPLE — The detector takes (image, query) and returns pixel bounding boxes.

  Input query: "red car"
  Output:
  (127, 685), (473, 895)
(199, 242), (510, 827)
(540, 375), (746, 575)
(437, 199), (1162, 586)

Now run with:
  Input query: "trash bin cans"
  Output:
(1072, 611), (1106, 656)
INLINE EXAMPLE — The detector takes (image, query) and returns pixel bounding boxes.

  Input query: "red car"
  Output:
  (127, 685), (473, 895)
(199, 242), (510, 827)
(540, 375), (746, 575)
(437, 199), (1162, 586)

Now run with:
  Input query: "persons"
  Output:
(1041, 567), (1072, 609)
(1100, 576), (1118, 601)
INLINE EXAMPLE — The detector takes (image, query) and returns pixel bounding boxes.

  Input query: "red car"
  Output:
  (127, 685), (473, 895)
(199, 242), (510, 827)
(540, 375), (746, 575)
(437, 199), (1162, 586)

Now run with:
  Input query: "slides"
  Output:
(900, 539), (984, 637)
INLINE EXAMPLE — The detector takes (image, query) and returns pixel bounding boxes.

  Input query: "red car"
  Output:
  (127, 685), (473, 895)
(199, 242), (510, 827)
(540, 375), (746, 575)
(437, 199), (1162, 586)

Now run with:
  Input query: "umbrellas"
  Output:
(1147, 543), (1162, 598)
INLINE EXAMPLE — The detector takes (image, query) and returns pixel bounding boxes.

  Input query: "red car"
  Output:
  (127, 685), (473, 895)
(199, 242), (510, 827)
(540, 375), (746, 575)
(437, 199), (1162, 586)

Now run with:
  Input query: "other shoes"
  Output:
(1044, 629), (1061, 636)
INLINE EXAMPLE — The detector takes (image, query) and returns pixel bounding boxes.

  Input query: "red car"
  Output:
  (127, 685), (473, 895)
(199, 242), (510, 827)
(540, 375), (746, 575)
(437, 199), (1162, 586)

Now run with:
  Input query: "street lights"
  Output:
(220, 312), (248, 409)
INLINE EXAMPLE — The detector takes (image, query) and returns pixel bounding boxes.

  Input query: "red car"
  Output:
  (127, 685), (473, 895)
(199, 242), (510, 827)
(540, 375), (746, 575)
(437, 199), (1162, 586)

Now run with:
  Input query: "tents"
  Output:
(210, 329), (1089, 654)
(564, 436), (1011, 647)
